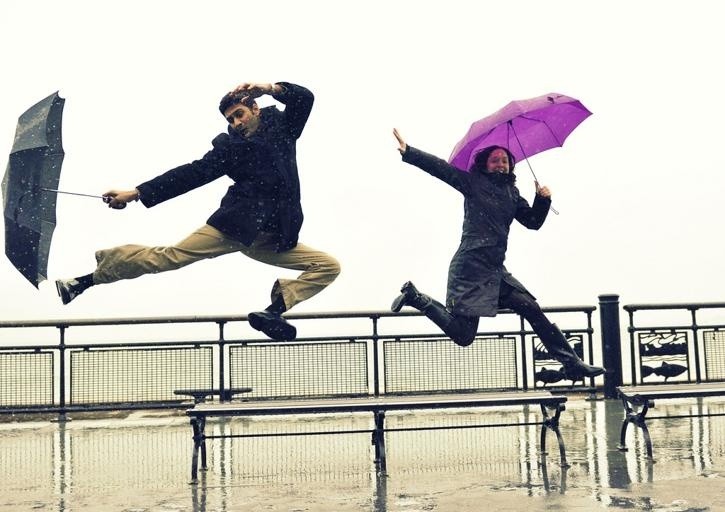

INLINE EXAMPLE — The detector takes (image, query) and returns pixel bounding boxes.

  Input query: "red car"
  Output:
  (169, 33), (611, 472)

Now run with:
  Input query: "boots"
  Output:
(390, 280), (468, 333)
(536, 317), (606, 382)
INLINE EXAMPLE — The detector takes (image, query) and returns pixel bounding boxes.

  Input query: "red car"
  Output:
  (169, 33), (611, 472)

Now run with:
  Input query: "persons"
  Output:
(54, 79), (341, 344)
(389, 125), (607, 381)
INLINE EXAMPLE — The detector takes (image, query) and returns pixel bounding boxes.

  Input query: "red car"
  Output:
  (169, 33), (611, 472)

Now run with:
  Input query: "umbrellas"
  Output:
(447, 91), (594, 199)
(0, 89), (128, 290)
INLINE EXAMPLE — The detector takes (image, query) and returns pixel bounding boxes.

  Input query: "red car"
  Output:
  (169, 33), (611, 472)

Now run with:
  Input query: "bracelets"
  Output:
(268, 81), (276, 95)
(135, 189), (140, 202)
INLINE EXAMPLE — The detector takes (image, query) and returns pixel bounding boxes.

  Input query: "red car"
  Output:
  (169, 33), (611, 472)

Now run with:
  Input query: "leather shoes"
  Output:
(54, 278), (81, 306)
(247, 309), (298, 343)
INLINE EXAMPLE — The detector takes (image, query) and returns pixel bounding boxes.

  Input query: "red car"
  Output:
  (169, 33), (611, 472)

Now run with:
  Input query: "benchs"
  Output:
(183, 388), (573, 486)
(617, 380), (725, 463)
